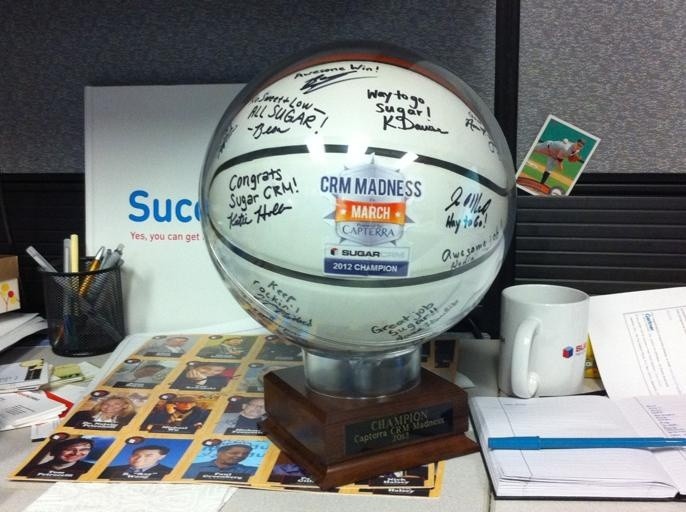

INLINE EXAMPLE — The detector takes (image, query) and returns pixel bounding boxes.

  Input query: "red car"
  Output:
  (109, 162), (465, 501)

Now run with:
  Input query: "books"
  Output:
(0, 313), (48, 352)
(466, 394), (686, 502)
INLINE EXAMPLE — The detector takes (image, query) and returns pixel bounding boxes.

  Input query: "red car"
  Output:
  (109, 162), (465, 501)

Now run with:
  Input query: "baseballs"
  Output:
(564, 138), (568, 144)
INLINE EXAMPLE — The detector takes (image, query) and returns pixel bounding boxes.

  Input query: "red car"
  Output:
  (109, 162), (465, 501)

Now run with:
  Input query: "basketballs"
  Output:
(202, 60), (509, 354)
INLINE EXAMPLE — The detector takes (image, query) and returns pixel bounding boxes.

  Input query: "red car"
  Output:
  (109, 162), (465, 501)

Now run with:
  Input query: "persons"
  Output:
(534, 139), (585, 184)
(67, 397), (134, 426)
(241, 366), (287, 388)
(198, 338), (248, 358)
(143, 397), (208, 428)
(215, 398), (266, 433)
(182, 444), (257, 478)
(260, 344), (302, 360)
(31, 438), (94, 477)
(173, 363), (228, 390)
(138, 338), (188, 357)
(106, 365), (164, 385)
(275, 463), (303, 476)
(379, 470), (422, 478)
(98, 446), (172, 479)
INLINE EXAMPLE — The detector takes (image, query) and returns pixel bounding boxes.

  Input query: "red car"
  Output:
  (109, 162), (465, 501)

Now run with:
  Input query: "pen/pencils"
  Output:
(26, 233), (125, 357)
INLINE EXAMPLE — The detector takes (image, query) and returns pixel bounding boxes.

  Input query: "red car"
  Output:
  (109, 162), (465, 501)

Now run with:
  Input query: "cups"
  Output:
(498, 284), (590, 399)
